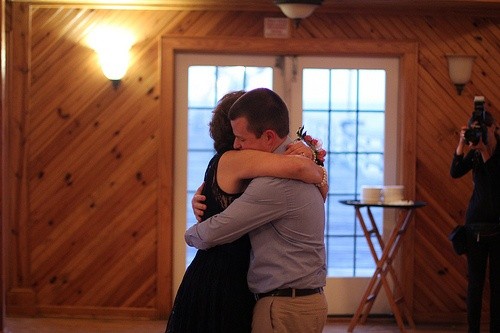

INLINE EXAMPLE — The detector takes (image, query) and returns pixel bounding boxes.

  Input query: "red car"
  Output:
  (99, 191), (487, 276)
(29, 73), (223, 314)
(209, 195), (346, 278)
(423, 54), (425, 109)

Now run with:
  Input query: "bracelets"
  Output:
(315, 164), (327, 188)
(311, 147), (317, 163)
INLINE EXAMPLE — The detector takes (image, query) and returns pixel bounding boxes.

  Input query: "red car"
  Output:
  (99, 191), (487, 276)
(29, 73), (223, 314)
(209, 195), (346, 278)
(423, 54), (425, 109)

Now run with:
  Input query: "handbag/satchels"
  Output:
(449, 224), (468, 256)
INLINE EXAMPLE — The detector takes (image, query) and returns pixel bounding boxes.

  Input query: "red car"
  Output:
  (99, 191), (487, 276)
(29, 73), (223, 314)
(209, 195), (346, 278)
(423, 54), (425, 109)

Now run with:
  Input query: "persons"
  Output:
(184, 88), (329, 332)
(449, 111), (500, 333)
(165, 90), (329, 333)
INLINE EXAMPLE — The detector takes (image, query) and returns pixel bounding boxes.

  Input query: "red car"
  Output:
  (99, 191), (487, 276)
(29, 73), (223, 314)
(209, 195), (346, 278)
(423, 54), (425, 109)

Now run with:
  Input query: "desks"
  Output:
(341, 200), (425, 333)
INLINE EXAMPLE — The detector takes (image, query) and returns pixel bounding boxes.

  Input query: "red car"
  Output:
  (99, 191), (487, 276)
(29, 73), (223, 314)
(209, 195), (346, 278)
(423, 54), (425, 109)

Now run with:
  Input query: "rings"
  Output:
(303, 152), (305, 156)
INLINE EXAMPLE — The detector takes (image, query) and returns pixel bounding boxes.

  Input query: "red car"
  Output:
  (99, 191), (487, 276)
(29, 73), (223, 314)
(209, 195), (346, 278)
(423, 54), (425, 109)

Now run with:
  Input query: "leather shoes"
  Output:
(255, 286), (324, 301)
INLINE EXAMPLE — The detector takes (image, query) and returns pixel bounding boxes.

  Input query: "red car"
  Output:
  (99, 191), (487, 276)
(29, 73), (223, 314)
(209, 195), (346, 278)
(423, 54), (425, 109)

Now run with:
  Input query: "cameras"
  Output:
(464, 96), (493, 147)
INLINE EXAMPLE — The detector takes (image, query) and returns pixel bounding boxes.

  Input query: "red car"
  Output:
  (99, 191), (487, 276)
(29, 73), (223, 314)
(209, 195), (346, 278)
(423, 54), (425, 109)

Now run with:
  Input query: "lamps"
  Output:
(445, 53), (477, 95)
(272, 0), (321, 29)
(98, 45), (131, 89)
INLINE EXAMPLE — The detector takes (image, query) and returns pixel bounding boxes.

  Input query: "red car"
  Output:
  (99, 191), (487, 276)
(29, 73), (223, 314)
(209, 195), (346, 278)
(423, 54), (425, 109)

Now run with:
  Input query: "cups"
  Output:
(362, 187), (382, 204)
(382, 186), (404, 204)
